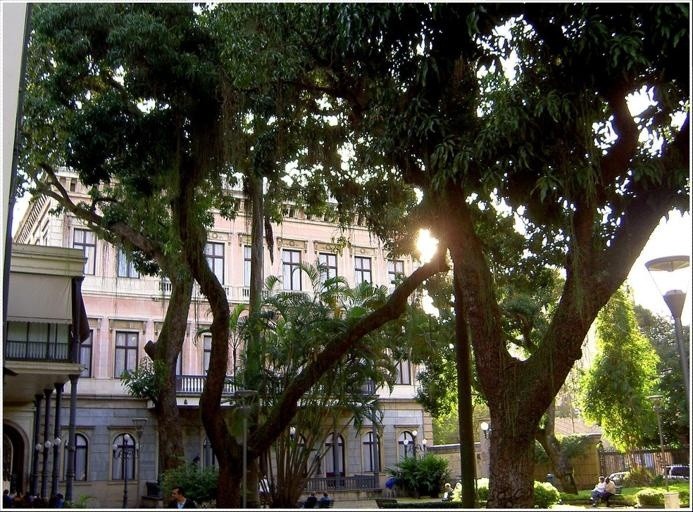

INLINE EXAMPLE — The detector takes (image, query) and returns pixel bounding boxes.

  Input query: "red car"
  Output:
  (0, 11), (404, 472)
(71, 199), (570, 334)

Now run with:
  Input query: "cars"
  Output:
(603, 470), (647, 487)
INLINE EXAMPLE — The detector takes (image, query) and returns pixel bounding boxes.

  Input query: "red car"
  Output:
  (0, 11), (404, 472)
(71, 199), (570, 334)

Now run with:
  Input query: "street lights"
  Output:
(480, 420), (493, 443)
(287, 424), (296, 501)
(401, 427), (427, 461)
(644, 393), (671, 493)
(643, 253), (688, 412)
(110, 432), (140, 508)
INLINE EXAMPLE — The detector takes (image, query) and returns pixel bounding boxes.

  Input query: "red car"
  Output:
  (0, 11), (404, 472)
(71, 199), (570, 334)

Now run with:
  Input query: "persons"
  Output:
(2, 487), (64, 508)
(167, 486), (197, 508)
(303, 491), (317, 508)
(192, 456), (200, 473)
(257, 473), (271, 508)
(443, 482), (454, 500)
(318, 491), (332, 508)
(603, 477), (616, 506)
(589, 476), (607, 506)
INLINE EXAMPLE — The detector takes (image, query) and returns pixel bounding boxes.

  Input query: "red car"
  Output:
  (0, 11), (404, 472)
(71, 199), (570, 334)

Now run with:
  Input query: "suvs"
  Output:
(659, 463), (689, 482)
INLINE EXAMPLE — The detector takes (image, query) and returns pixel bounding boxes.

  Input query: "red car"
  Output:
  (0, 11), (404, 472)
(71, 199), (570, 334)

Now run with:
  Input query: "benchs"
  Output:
(304, 500), (334, 508)
(609, 486), (628, 507)
(376, 499), (400, 509)
